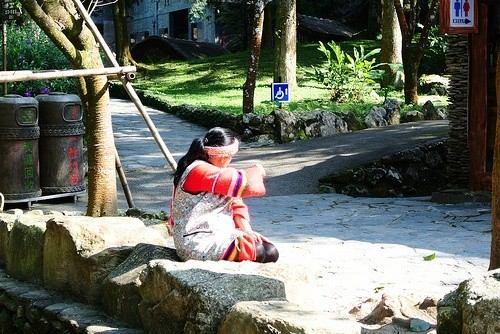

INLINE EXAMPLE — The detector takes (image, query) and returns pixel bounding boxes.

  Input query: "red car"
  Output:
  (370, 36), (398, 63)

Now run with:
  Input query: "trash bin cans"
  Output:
(0, 94), (42, 200)
(35, 92), (85, 193)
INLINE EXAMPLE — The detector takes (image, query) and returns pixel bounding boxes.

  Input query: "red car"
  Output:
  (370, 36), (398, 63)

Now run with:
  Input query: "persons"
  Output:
(171, 126), (279, 263)
